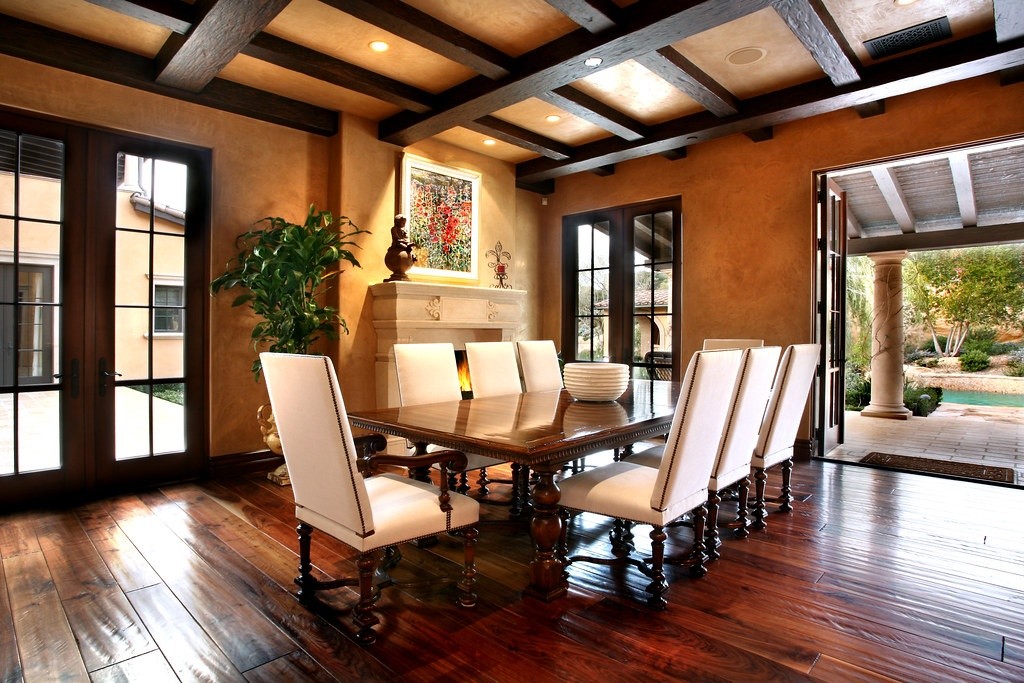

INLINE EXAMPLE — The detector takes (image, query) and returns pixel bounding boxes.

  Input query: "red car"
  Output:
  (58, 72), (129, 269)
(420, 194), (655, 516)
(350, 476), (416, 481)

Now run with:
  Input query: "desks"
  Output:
(346, 378), (685, 605)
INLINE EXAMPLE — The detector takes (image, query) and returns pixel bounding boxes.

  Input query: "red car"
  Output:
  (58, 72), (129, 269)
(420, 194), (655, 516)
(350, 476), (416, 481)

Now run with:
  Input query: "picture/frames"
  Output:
(398, 150), (483, 285)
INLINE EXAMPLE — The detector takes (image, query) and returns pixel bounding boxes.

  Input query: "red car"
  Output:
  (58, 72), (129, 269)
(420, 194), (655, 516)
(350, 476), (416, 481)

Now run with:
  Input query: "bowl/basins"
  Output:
(563, 363), (630, 401)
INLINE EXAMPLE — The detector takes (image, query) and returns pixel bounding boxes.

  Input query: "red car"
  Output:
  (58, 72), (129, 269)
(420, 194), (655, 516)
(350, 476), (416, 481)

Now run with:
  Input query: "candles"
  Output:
(497, 262), (505, 273)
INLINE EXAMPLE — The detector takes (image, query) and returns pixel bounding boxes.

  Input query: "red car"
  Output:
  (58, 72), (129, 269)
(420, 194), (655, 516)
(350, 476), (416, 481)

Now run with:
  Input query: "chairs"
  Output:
(665, 346), (785, 561)
(556, 338), (743, 611)
(259, 352), (481, 648)
(394, 337), (586, 538)
(749, 344), (822, 529)
(703, 338), (764, 353)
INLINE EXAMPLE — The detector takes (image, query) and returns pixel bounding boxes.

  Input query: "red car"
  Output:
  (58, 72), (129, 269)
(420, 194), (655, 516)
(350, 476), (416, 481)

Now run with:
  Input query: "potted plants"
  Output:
(205, 201), (375, 488)
